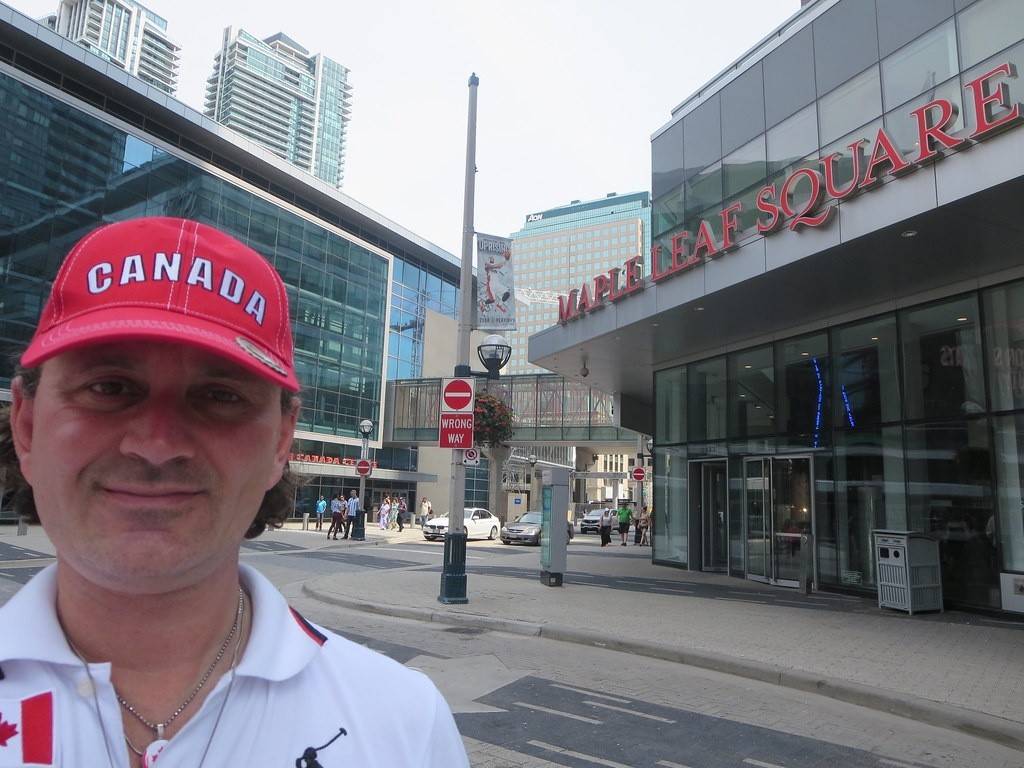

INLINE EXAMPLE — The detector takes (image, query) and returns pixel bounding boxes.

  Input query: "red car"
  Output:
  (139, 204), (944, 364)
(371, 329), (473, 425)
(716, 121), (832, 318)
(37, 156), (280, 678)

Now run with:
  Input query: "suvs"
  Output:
(581, 508), (621, 535)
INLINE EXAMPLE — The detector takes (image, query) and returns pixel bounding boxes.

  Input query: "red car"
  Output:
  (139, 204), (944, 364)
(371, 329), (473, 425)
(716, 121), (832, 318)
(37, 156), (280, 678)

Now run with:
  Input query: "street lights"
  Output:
(528, 455), (537, 512)
(437, 334), (512, 605)
(348, 419), (374, 541)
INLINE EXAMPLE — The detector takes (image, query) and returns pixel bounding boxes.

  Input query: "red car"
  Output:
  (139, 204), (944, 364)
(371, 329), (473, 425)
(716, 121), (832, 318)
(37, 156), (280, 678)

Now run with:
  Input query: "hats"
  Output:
(19, 217), (299, 393)
(605, 508), (610, 511)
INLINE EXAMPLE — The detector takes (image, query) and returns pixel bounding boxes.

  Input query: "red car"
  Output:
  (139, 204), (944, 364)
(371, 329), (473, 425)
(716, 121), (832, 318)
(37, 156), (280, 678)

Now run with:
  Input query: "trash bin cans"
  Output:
(872, 529), (944, 616)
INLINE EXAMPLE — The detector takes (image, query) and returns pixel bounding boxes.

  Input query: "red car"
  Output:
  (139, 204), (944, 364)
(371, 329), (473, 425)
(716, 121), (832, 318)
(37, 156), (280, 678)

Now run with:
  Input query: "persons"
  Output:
(301, 498), (310, 530)
(635, 507), (650, 546)
(600, 508), (612, 547)
(616, 503), (633, 546)
(583, 507), (588, 516)
(315, 495), (326, 531)
(0, 217), (468, 767)
(781, 520), (800, 554)
(326, 490), (359, 540)
(480, 257), (510, 313)
(420, 498), (431, 527)
(376, 496), (406, 533)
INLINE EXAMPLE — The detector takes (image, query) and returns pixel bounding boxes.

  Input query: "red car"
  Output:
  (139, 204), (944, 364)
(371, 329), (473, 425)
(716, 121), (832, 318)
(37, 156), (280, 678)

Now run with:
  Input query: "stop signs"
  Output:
(355, 458), (372, 476)
(441, 378), (476, 413)
(632, 467), (646, 482)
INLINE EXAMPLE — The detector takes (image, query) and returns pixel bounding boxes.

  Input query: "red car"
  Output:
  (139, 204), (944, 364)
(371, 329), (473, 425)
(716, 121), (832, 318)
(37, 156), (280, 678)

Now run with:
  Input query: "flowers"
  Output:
(472, 393), (514, 447)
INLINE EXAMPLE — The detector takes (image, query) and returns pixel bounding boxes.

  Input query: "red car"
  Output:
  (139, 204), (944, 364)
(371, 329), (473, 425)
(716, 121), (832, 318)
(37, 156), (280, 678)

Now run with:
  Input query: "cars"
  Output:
(500, 511), (575, 547)
(422, 507), (501, 543)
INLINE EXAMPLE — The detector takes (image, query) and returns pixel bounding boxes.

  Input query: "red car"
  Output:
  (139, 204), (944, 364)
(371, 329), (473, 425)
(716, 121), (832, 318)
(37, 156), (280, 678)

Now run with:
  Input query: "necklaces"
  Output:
(66, 587), (242, 768)
(125, 592), (242, 756)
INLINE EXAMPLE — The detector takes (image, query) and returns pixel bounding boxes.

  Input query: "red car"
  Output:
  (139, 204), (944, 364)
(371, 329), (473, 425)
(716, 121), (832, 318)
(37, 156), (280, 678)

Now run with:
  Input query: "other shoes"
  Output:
(327, 536), (331, 539)
(341, 536), (347, 539)
(333, 537), (338, 540)
(381, 525), (404, 532)
(621, 543), (626, 546)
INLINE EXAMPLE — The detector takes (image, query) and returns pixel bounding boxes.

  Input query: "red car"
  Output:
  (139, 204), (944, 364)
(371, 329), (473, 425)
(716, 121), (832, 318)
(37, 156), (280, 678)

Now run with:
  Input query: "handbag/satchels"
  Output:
(427, 502), (433, 514)
